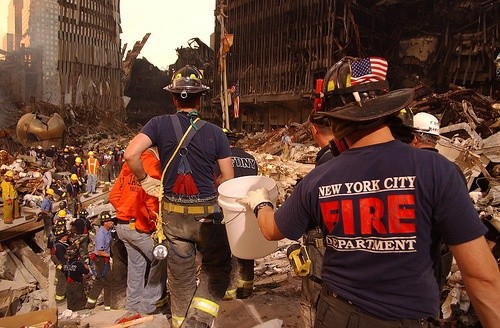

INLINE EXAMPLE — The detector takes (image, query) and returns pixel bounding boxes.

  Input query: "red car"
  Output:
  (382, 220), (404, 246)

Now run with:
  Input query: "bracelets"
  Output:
(137, 173), (148, 181)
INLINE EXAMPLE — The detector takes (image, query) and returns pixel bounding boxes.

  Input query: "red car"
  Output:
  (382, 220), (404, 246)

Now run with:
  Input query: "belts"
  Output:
(163, 201), (221, 214)
(118, 219), (130, 225)
(323, 284), (425, 322)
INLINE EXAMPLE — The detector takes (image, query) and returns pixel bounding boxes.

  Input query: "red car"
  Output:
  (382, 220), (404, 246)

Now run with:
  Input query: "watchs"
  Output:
(253, 202), (274, 218)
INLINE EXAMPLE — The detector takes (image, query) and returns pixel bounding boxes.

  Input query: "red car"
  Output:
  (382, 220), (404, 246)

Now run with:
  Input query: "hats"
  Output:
(163, 64), (210, 99)
(315, 56), (413, 121)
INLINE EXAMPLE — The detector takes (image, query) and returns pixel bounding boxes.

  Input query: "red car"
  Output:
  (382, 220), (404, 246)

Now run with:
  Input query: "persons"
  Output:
(410, 111), (468, 280)
(0, 143), (125, 238)
(48, 209), (95, 312)
(301, 109), (335, 328)
(212, 128), (258, 300)
(85, 210), (118, 311)
(109, 146), (167, 315)
(236, 57), (500, 328)
(282, 130), (292, 162)
(107, 219), (129, 311)
(124, 65), (234, 328)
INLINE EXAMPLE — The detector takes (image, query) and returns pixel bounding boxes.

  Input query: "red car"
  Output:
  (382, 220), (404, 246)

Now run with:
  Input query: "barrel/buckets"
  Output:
(218, 176), (278, 259)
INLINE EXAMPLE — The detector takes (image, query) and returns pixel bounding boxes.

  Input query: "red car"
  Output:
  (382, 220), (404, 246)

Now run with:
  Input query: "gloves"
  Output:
(138, 173), (164, 198)
(236, 187), (274, 218)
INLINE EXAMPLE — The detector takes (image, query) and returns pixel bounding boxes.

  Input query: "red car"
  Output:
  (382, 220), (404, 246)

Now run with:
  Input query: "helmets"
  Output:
(36, 143), (81, 156)
(413, 112), (440, 136)
(98, 211), (113, 222)
(76, 208), (89, 216)
(88, 151), (95, 155)
(4, 171), (14, 177)
(46, 188), (55, 196)
(56, 220), (68, 242)
(222, 127), (235, 137)
(58, 210), (66, 217)
(75, 156), (82, 162)
(70, 173), (79, 181)
(64, 246), (78, 260)
